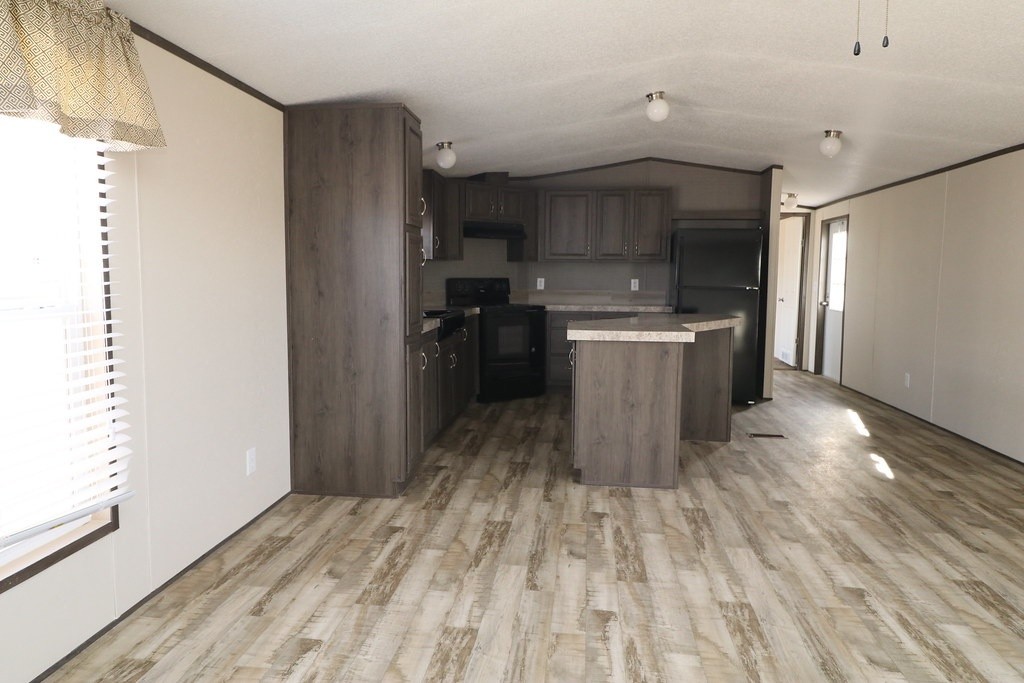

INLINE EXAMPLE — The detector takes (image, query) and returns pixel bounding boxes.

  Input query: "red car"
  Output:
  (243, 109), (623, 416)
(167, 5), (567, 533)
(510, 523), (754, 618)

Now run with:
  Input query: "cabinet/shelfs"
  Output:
(544, 191), (592, 259)
(434, 171), (461, 258)
(465, 183), (529, 224)
(422, 337), (440, 451)
(597, 188), (667, 260)
(438, 321), (478, 431)
(285, 103), (427, 494)
(549, 311), (741, 491)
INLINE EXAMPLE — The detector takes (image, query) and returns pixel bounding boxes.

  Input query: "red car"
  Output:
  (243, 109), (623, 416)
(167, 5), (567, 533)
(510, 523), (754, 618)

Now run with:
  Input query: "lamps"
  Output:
(436, 141), (455, 169)
(821, 130), (841, 157)
(646, 90), (669, 122)
(784, 193), (798, 209)
